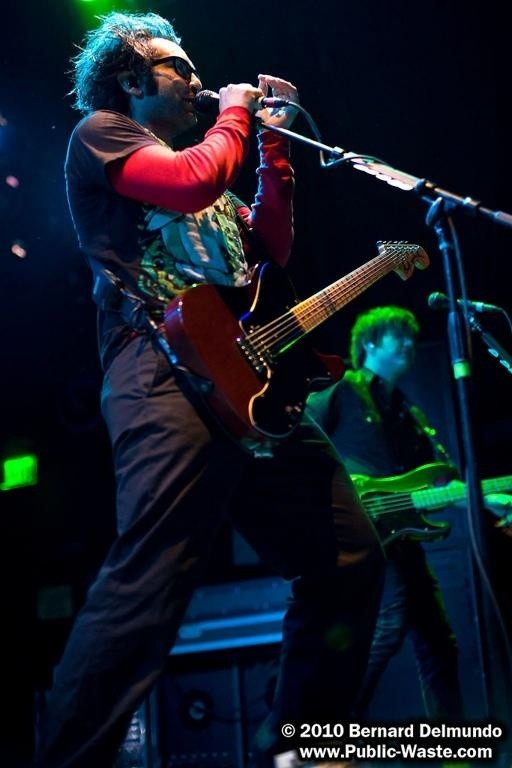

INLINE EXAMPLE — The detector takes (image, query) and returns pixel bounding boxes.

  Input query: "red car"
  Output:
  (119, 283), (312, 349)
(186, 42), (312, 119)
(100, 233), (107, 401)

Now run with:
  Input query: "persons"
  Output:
(303, 303), (512, 767)
(19, 7), (388, 767)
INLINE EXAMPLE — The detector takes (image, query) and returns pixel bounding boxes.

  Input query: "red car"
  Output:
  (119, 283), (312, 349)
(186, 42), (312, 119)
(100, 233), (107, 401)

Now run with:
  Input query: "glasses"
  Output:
(147, 56), (200, 79)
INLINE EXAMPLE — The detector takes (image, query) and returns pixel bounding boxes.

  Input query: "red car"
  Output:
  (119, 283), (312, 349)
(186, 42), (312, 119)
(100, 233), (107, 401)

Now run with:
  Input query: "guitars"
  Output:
(166, 240), (429, 440)
(349, 462), (512, 545)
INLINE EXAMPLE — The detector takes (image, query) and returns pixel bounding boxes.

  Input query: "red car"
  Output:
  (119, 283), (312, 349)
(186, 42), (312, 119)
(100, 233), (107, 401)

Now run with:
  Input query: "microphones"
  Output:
(192, 90), (289, 113)
(428, 292), (502, 315)
